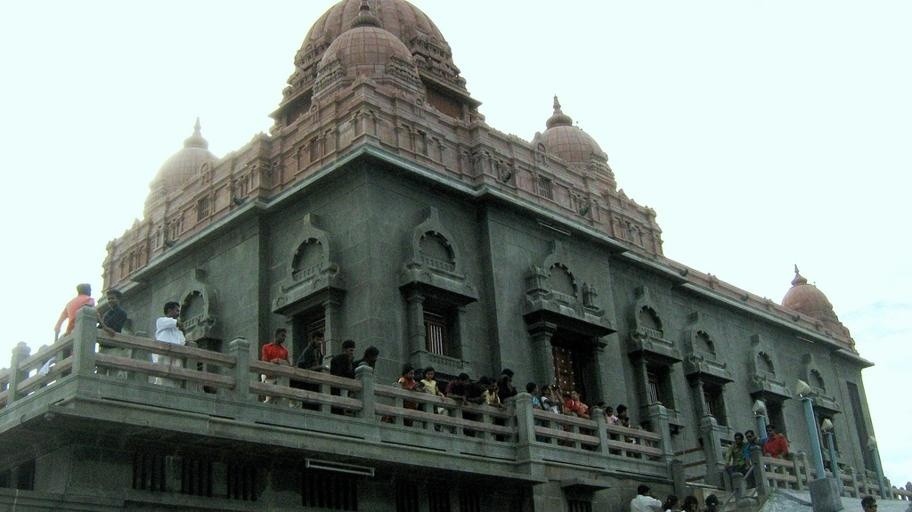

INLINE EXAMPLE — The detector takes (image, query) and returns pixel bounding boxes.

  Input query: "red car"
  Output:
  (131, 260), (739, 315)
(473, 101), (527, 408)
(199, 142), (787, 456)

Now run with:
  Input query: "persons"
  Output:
(735, 429), (785, 489)
(720, 432), (752, 492)
(398, 365), (632, 457)
(53, 281), (118, 343)
(329, 339), (358, 415)
(355, 344), (378, 372)
(259, 327), (294, 403)
(861, 495), (878, 512)
(628, 482), (721, 512)
(151, 301), (189, 388)
(295, 332), (325, 411)
(99, 288), (127, 379)
(750, 424), (788, 490)
